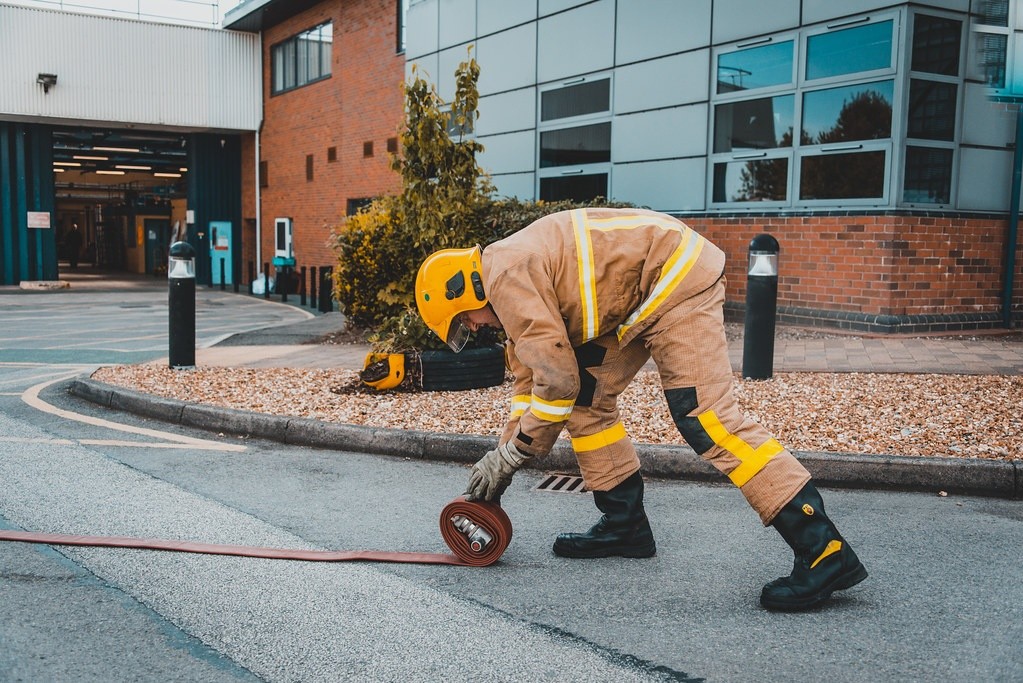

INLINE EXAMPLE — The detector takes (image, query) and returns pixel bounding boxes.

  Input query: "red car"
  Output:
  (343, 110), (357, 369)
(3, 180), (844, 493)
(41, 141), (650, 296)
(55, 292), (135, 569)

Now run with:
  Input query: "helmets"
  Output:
(362, 353), (404, 391)
(415, 243), (491, 353)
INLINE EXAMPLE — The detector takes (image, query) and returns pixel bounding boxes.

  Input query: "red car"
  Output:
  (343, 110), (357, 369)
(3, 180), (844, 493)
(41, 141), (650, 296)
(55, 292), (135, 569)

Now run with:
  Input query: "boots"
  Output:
(553, 469), (656, 560)
(760, 479), (869, 613)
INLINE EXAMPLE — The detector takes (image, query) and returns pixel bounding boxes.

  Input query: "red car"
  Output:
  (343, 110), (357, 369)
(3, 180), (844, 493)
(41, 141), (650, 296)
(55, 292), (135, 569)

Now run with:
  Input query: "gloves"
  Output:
(467, 443), (520, 507)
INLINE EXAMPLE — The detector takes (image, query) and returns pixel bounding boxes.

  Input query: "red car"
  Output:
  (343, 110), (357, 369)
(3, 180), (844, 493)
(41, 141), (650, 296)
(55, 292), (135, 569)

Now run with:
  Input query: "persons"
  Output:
(415, 209), (869, 611)
